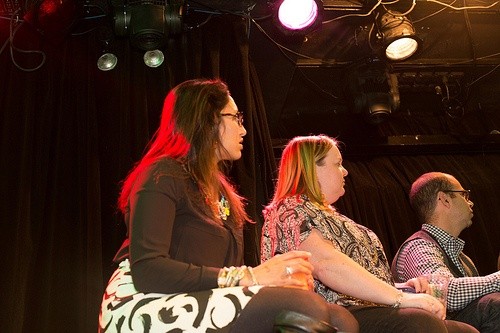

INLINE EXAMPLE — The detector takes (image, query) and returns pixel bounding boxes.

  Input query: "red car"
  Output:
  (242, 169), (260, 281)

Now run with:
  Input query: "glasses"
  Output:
(438, 189), (471, 202)
(220, 111), (245, 127)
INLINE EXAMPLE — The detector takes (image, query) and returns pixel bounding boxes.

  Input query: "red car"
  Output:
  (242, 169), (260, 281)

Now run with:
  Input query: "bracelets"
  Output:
(218, 265), (258, 288)
(393, 289), (403, 308)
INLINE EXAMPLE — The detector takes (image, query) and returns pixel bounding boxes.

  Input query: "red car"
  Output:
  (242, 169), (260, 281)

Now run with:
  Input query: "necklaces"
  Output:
(213, 195), (230, 221)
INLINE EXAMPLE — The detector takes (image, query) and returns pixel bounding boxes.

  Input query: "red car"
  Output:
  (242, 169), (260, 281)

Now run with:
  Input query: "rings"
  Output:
(286, 267), (292, 275)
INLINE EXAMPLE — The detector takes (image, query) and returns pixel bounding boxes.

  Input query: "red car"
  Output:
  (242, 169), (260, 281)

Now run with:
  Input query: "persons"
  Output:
(392, 172), (500, 333)
(98, 80), (361, 333)
(261, 136), (480, 333)
